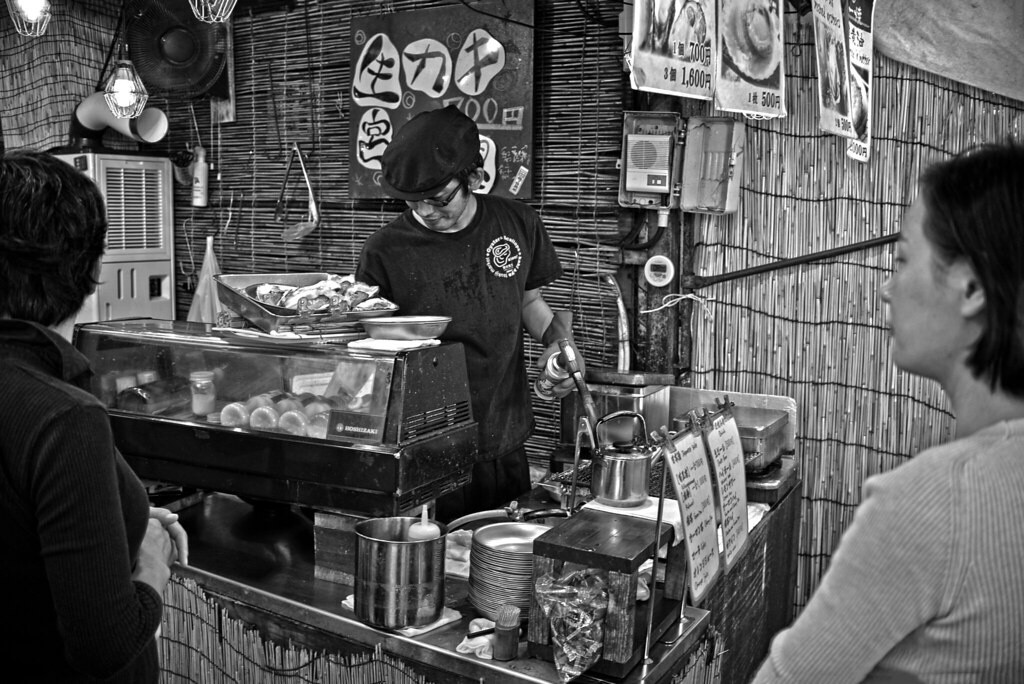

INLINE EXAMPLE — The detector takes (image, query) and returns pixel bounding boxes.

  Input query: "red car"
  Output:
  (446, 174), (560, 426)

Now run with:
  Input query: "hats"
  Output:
(381, 105), (479, 201)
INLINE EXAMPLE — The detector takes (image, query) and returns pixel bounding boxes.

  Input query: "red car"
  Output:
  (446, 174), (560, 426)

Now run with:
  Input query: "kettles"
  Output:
(590, 410), (662, 508)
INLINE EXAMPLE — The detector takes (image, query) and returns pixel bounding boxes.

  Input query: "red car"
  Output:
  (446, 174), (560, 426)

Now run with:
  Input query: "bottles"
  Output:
(407, 505), (440, 542)
(220, 389), (372, 439)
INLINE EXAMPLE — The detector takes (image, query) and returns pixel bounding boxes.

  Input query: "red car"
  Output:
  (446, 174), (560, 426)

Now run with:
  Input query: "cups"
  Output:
(190, 372), (215, 417)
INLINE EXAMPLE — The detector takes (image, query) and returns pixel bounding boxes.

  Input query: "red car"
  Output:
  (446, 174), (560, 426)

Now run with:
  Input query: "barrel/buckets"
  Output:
(352, 517), (449, 630)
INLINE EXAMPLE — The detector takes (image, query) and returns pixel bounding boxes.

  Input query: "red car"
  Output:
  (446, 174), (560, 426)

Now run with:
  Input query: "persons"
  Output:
(0, 147), (189, 684)
(745, 135), (1024, 684)
(320, 105), (585, 525)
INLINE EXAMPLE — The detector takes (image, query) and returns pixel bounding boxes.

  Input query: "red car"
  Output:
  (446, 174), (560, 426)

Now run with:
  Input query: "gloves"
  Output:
(538, 311), (586, 399)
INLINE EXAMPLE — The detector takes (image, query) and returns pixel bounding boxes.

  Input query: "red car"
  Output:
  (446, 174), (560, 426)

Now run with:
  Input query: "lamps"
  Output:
(105, 0), (148, 118)
(5, 0), (54, 36)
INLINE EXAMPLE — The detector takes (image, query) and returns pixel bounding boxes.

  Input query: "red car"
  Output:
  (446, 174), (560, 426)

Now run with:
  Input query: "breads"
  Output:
(220, 391), (346, 439)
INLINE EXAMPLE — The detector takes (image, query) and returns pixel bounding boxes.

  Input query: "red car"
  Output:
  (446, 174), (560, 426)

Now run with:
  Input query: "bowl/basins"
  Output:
(468, 522), (554, 622)
(359, 315), (452, 340)
(245, 283), (298, 314)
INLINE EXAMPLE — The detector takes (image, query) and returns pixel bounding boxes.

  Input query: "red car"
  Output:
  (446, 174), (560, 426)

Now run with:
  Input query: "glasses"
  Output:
(407, 182), (464, 207)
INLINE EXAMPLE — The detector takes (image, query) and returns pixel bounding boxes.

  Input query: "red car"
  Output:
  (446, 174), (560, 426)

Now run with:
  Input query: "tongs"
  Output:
(275, 142), (319, 222)
